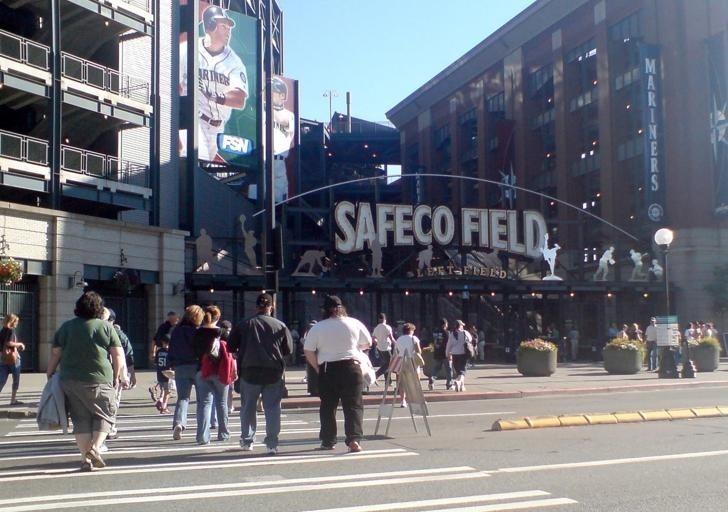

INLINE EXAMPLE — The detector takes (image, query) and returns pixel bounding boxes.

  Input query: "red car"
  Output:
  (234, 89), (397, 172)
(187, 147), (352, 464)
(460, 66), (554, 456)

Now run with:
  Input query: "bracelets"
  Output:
(217, 92), (225, 105)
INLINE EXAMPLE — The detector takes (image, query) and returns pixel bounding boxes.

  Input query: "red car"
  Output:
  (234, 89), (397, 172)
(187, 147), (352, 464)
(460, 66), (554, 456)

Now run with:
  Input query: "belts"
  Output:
(197, 113), (223, 126)
(272, 156), (284, 160)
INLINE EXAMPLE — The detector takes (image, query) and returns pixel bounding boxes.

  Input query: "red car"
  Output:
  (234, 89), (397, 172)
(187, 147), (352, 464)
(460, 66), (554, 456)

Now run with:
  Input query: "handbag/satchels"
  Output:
(0, 345), (16, 366)
(414, 352), (425, 367)
(463, 340), (475, 359)
(207, 336), (222, 366)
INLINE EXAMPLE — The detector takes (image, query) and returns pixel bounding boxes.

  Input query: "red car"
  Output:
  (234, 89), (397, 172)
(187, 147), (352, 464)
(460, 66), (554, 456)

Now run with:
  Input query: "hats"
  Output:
(456, 320), (466, 327)
(255, 293), (273, 307)
(317, 295), (343, 308)
(376, 312), (386, 320)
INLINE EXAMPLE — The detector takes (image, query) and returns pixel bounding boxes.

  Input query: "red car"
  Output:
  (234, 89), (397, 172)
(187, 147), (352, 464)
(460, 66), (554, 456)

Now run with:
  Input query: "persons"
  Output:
(392, 322), (423, 408)
(240, 214), (258, 272)
(471, 317), (719, 371)
(0, 313), (25, 405)
(196, 228), (215, 273)
(426, 317), (454, 393)
(46, 292), (137, 471)
(539, 233), (561, 277)
(180, 6), (248, 169)
(446, 319), (476, 392)
(649, 259), (664, 278)
(300, 295), (373, 451)
(148, 294), (293, 456)
(294, 250), (328, 276)
(594, 247), (617, 280)
(371, 238), (383, 276)
(262, 78), (295, 206)
(629, 247), (647, 281)
(417, 244), (434, 271)
(285, 324), (299, 367)
(371, 312), (399, 385)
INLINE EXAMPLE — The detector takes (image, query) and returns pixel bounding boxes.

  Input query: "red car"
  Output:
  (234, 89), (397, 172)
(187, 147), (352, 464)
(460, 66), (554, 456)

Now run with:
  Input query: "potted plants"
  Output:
(0, 257), (24, 285)
(692, 337), (722, 371)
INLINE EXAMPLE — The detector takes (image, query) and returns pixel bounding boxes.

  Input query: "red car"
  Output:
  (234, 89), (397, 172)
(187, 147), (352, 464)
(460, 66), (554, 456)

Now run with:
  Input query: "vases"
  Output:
(515, 350), (557, 377)
(604, 344), (641, 374)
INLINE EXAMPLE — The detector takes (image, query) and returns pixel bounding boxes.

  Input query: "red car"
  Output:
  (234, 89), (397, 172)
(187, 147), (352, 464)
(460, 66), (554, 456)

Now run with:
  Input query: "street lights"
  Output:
(320, 88), (339, 134)
(654, 226), (680, 378)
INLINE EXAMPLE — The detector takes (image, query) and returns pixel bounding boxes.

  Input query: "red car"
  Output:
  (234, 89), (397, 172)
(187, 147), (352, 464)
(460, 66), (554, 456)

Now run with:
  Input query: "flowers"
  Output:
(518, 338), (556, 352)
(605, 337), (641, 352)
(113, 268), (139, 290)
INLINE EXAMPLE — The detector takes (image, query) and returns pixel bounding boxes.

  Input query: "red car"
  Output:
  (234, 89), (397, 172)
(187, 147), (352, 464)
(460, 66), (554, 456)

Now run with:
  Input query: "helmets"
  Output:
(271, 78), (288, 95)
(202, 5), (235, 31)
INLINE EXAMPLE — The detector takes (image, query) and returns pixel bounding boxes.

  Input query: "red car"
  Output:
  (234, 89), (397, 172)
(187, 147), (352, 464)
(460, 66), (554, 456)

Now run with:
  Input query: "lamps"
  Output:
(173, 279), (192, 296)
(68, 271), (88, 289)
